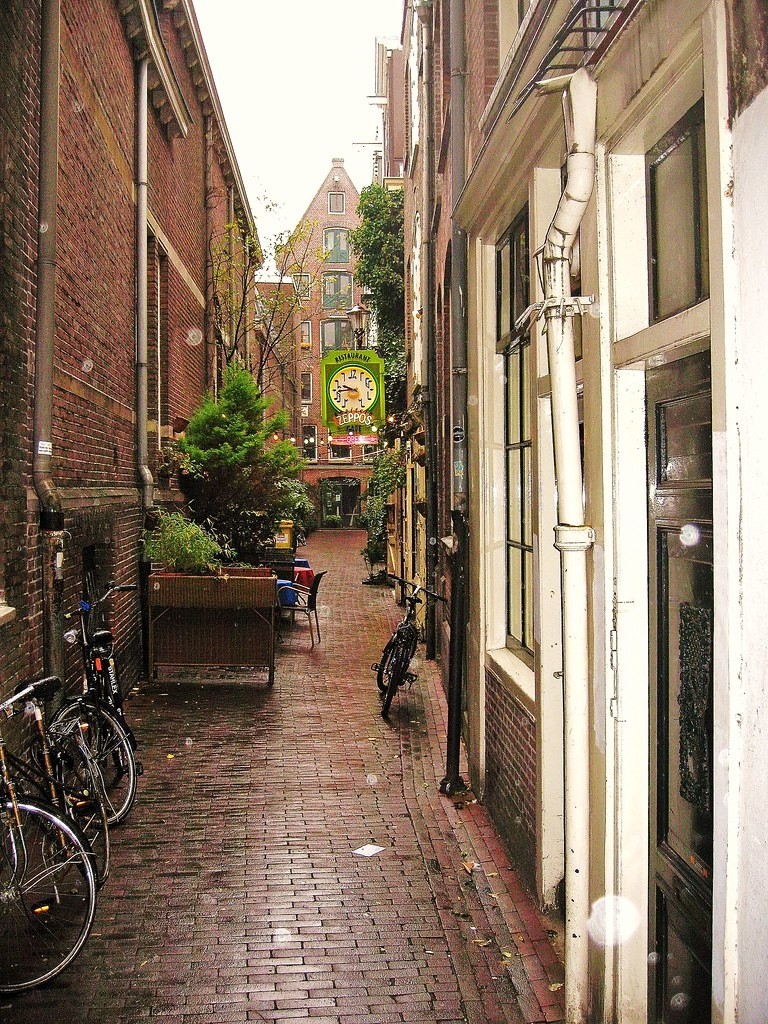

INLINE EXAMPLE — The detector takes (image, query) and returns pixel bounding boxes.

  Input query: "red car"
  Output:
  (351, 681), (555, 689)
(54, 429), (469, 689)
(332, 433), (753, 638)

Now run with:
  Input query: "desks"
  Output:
(277, 555), (315, 604)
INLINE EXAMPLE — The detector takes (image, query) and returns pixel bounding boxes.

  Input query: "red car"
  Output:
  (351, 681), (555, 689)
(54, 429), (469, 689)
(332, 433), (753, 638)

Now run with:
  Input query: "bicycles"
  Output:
(0, 581), (145, 994)
(370, 573), (448, 719)
(292, 529), (308, 547)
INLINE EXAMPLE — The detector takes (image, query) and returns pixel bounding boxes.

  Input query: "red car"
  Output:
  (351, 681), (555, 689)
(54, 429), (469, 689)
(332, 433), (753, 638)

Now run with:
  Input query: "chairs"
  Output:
(258, 546), (296, 622)
(277, 570), (328, 648)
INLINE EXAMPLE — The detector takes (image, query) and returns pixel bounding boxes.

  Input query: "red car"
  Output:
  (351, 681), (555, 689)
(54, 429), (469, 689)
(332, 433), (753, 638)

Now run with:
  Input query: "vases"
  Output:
(413, 432), (425, 447)
(172, 415), (191, 433)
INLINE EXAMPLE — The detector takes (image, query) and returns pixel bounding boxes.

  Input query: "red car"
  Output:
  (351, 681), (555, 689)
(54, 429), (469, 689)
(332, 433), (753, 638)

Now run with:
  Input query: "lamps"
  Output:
(345, 303), (387, 360)
(412, 306), (423, 320)
(333, 173), (340, 184)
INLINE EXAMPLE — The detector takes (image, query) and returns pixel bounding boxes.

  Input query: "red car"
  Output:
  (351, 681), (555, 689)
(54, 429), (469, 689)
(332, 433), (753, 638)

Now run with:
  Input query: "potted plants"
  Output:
(414, 493), (428, 517)
(411, 448), (426, 468)
(136, 499), (279, 688)
